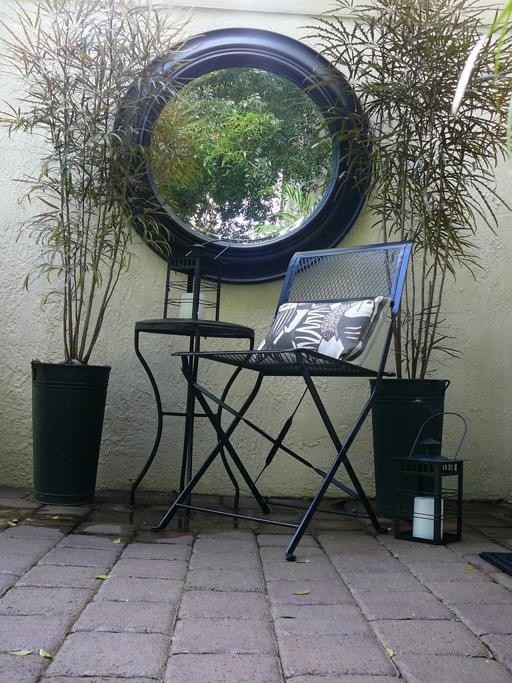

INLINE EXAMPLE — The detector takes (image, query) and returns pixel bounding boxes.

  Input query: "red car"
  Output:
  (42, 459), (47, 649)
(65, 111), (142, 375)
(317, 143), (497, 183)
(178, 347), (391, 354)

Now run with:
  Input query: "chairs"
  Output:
(150, 239), (415, 562)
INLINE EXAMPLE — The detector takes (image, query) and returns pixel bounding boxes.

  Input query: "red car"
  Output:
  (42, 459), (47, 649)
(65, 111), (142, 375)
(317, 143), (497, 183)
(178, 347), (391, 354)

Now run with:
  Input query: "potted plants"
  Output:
(291, 0), (512, 520)
(1, 0), (209, 504)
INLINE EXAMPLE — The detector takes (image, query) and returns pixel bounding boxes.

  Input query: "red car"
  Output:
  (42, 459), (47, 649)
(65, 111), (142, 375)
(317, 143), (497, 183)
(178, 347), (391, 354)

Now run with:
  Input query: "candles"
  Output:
(412, 495), (447, 541)
(177, 291), (203, 320)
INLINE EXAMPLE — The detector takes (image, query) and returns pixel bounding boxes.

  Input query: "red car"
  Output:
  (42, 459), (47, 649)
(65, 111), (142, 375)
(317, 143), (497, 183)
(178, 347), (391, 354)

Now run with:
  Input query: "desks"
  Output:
(131, 318), (255, 507)
